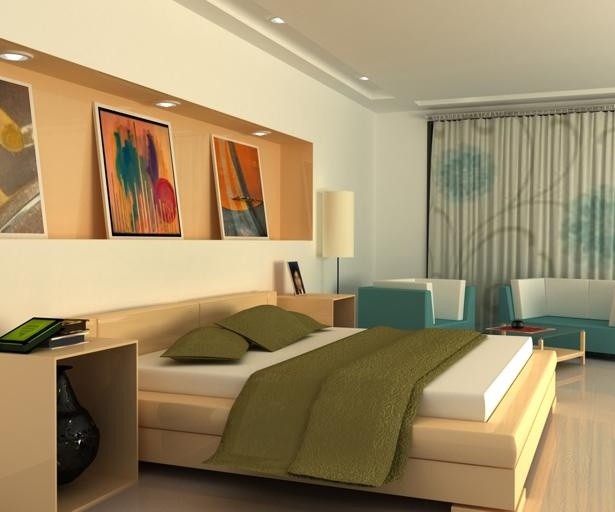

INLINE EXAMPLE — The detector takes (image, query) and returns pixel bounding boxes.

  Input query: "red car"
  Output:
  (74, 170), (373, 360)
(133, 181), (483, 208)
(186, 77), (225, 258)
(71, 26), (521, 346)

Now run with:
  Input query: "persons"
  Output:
(291, 267), (305, 296)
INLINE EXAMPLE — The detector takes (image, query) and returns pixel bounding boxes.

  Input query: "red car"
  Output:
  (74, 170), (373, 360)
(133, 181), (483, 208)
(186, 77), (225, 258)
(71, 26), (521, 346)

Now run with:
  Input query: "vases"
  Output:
(56, 365), (100, 486)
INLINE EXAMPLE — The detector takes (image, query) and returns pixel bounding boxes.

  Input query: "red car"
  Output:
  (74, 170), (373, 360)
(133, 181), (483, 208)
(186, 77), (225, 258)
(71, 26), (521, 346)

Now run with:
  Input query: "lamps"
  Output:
(323, 190), (354, 293)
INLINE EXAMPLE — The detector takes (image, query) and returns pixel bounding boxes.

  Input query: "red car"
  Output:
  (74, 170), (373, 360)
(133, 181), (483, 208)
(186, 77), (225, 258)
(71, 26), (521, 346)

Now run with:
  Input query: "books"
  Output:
(0, 315), (92, 352)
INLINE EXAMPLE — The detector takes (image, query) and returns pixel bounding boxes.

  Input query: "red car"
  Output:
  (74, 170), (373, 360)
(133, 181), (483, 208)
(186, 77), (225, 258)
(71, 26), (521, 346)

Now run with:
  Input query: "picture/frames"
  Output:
(93, 103), (183, 239)
(211, 135), (269, 240)
(0, 76), (49, 239)
(287, 259), (307, 296)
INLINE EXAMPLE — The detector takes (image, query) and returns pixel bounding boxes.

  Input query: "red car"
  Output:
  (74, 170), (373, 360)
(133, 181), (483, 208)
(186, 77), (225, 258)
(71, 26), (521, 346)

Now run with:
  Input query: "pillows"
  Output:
(161, 327), (250, 360)
(290, 309), (333, 332)
(216, 305), (314, 352)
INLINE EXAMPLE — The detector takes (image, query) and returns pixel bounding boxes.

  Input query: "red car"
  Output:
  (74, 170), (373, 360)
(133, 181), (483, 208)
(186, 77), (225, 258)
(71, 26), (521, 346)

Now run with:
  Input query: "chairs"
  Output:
(358, 278), (476, 329)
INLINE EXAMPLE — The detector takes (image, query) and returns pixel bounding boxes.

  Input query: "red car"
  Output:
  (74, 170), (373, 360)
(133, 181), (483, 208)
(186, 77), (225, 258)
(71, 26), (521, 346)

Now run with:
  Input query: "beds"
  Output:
(75, 292), (558, 512)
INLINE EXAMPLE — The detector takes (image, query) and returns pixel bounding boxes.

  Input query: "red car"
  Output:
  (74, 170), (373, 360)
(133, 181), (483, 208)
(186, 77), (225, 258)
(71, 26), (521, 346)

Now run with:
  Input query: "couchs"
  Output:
(499, 278), (615, 357)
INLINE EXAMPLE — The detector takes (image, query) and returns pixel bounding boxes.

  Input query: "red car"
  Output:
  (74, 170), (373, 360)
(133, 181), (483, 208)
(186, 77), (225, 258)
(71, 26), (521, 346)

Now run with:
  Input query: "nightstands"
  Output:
(278, 293), (355, 329)
(0, 337), (140, 512)
